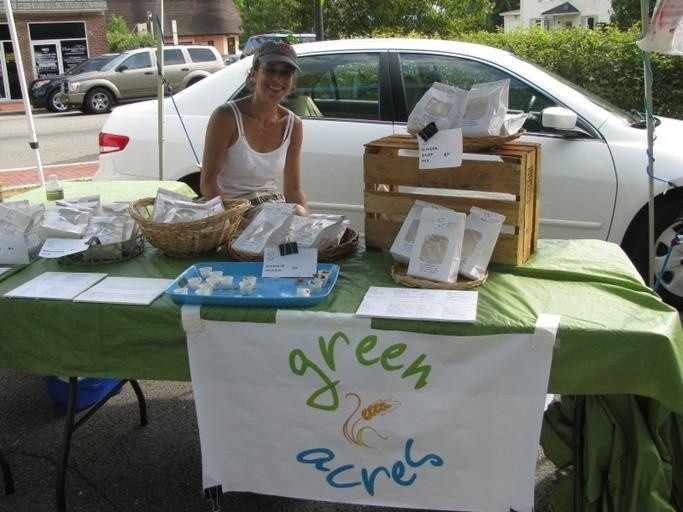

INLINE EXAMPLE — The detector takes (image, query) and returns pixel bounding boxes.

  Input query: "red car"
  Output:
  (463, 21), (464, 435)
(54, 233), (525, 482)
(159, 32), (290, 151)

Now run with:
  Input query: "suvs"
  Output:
(59, 44), (225, 114)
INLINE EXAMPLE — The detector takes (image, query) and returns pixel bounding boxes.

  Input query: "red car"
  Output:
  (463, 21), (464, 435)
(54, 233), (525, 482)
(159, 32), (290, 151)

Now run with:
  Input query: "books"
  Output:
(3, 271), (176, 307)
(354, 285), (477, 325)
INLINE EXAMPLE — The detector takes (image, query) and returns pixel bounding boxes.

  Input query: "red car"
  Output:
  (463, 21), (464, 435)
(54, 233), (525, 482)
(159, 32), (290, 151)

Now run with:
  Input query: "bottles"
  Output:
(46, 174), (64, 200)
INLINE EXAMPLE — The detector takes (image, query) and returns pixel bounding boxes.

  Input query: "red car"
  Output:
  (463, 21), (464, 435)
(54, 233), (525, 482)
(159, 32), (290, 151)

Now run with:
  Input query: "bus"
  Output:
(237, 34), (327, 60)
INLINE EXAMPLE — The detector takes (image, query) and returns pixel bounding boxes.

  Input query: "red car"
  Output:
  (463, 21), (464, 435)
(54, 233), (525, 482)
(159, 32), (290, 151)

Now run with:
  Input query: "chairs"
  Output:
(284, 70), (442, 122)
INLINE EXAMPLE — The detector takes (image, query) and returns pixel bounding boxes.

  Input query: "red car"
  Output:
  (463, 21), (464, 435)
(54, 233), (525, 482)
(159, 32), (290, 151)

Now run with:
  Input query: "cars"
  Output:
(221, 54), (237, 64)
(92, 34), (683, 320)
(26, 50), (120, 113)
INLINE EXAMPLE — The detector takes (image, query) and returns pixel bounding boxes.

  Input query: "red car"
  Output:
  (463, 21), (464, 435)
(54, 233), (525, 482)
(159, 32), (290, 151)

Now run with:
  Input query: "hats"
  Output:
(253, 41), (302, 73)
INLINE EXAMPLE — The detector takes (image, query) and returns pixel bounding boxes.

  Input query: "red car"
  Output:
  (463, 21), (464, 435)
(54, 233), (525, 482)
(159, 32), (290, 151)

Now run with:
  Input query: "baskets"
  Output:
(390, 261), (488, 289)
(54, 196), (359, 265)
(406, 124), (525, 150)
(226, 227), (358, 263)
(127, 198), (251, 258)
(54, 232), (146, 264)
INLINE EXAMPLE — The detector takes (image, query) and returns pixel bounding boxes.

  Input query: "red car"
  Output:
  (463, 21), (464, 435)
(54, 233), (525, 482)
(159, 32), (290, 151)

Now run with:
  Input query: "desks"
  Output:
(0, 240), (683, 512)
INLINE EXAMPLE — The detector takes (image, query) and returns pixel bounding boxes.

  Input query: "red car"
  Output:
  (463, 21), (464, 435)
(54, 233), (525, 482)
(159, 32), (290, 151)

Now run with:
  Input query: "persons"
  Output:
(200, 41), (309, 230)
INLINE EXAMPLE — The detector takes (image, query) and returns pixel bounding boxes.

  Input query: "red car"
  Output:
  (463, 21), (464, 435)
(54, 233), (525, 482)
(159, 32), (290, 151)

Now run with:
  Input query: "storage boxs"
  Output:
(363, 135), (542, 267)
